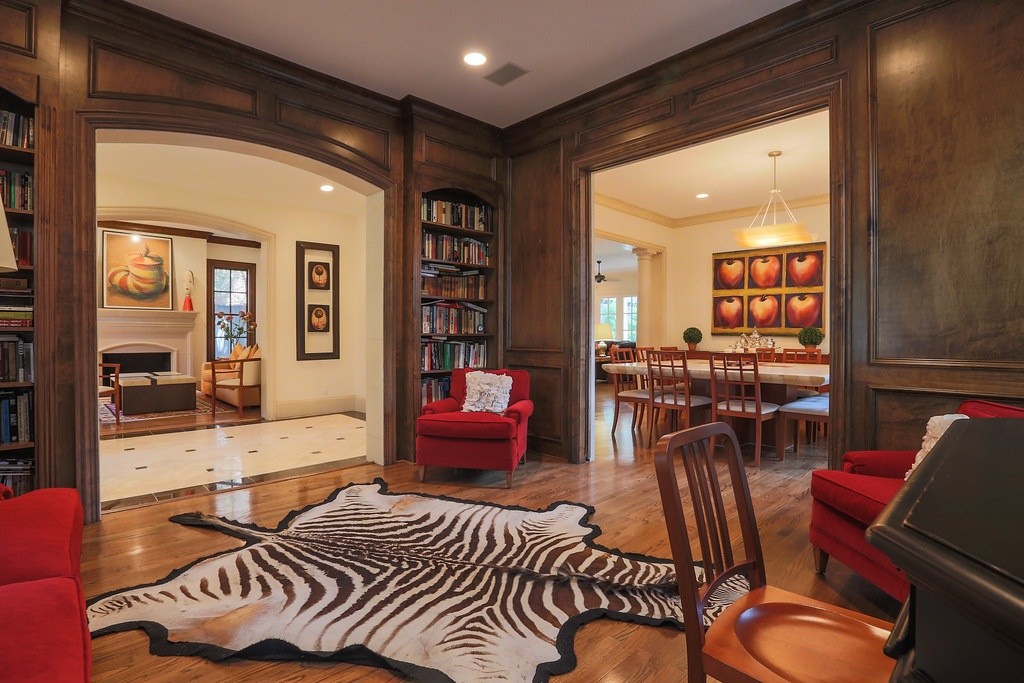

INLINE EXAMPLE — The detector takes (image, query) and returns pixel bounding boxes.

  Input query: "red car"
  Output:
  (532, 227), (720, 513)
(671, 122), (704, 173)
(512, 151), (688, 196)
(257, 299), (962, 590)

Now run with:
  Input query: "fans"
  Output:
(594, 261), (623, 283)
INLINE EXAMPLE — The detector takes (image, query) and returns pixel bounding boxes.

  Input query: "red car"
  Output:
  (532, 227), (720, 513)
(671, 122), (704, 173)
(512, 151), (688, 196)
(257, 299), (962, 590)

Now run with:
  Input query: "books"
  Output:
(0, 108), (36, 497)
(421, 198), (492, 403)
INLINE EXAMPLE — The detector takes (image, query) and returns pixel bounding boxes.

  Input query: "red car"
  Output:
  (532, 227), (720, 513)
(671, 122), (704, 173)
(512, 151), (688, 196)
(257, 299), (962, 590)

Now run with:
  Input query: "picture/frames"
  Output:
(102, 230), (173, 310)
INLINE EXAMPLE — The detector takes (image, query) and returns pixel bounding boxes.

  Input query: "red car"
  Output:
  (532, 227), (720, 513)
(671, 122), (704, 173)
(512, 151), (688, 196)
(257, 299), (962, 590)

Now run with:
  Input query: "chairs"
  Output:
(98, 363), (121, 425)
(612, 345), (1024, 683)
(211, 358), (261, 418)
(416, 368), (535, 487)
(0, 483), (91, 683)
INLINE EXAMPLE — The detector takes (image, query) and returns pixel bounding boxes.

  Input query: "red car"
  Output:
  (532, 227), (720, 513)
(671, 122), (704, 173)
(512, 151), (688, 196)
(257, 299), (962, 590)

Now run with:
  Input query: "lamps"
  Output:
(732, 149), (812, 249)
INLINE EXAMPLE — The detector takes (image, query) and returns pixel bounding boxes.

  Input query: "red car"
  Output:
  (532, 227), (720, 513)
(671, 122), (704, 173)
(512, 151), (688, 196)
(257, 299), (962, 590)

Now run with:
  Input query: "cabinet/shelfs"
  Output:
(0, 0), (58, 502)
(402, 114), (503, 466)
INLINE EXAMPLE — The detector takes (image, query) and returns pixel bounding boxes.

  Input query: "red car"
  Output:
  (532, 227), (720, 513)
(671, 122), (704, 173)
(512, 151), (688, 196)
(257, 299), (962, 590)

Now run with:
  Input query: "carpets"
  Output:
(85, 475), (750, 683)
(100, 397), (235, 424)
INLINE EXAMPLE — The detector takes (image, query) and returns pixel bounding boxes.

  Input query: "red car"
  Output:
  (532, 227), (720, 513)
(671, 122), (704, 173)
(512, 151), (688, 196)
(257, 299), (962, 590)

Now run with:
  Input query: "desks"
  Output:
(595, 356), (616, 384)
(601, 359), (829, 448)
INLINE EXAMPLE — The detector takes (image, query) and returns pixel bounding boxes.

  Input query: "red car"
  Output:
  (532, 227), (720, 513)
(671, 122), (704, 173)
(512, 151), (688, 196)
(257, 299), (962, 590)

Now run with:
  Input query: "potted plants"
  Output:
(683, 327), (702, 350)
(797, 326), (823, 354)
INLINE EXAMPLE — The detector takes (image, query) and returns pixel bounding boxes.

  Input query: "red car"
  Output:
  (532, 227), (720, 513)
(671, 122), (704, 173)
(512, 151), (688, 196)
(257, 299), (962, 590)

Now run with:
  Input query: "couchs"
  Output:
(296, 240), (340, 361)
(595, 341), (636, 381)
(201, 361), (261, 407)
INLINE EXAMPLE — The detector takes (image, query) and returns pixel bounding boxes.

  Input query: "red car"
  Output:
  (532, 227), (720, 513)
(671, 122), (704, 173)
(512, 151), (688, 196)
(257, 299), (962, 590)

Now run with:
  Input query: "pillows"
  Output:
(905, 414), (970, 481)
(460, 371), (513, 418)
(229, 343), (261, 369)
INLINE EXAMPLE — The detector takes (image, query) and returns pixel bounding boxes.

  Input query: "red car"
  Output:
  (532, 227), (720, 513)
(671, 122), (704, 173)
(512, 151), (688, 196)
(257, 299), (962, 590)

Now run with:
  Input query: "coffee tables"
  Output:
(109, 371), (197, 415)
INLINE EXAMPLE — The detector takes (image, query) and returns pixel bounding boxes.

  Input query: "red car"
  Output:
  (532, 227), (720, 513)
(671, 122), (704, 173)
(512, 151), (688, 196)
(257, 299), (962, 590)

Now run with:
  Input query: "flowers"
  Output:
(216, 311), (257, 347)
